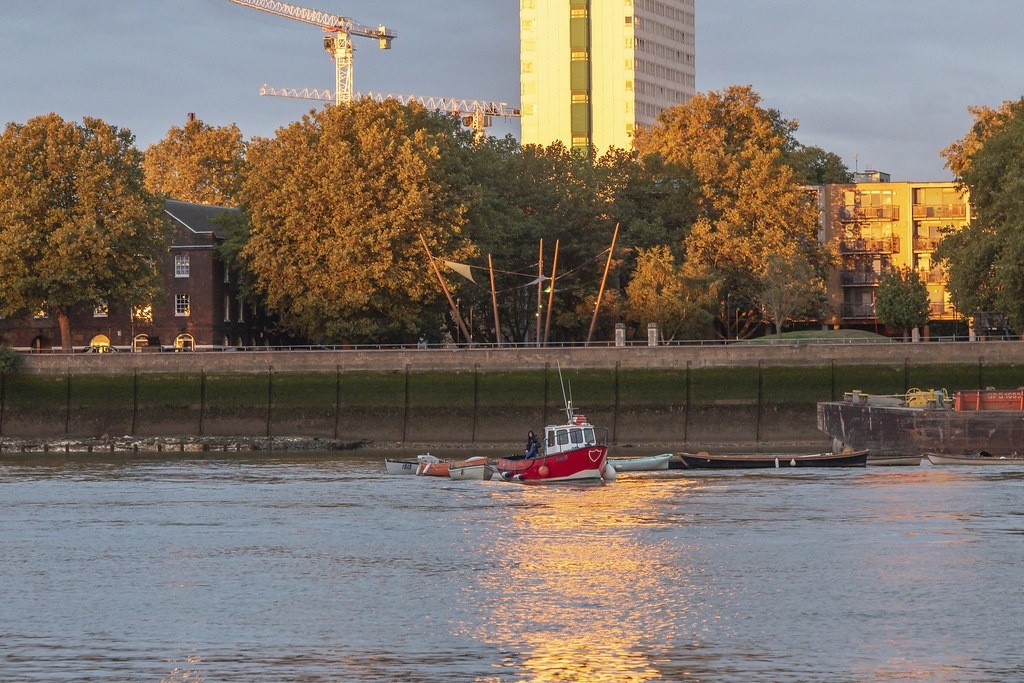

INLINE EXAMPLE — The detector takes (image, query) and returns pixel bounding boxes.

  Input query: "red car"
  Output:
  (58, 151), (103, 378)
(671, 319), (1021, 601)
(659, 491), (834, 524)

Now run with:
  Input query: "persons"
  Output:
(831, 314), (840, 333)
(525, 431), (541, 459)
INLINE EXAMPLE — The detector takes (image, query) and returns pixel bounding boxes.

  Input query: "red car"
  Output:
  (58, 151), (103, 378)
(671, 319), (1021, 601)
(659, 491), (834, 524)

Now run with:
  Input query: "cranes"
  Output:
(258, 81), (522, 149)
(227, 0), (398, 108)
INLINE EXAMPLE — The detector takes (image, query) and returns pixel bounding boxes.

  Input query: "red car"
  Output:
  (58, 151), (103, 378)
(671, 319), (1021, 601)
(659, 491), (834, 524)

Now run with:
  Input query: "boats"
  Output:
(678, 448), (870, 470)
(817, 386), (1024, 462)
(385, 453), (494, 482)
(866, 454), (923, 466)
(606, 453), (674, 472)
(494, 356), (617, 484)
(927, 453), (1024, 465)
